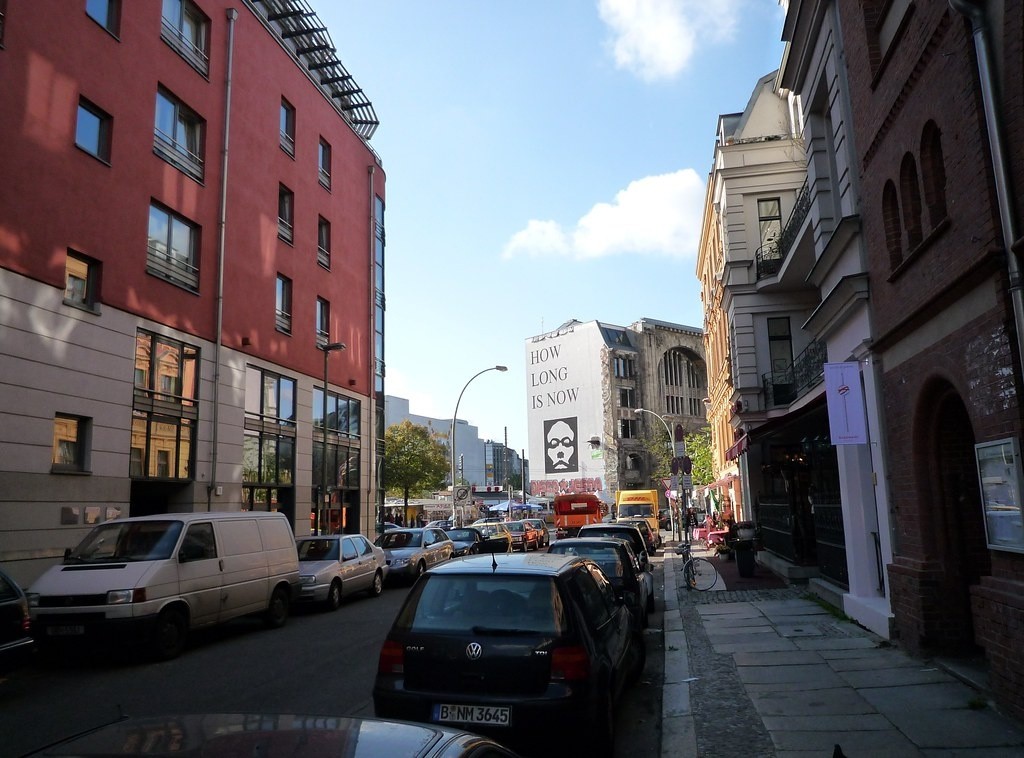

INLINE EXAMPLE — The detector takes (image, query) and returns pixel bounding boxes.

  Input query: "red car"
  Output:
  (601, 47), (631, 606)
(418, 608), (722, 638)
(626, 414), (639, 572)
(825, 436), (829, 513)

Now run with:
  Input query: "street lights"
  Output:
(633, 407), (680, 542)
(321, 341), (347, 535)
(450, 365), (508, 528)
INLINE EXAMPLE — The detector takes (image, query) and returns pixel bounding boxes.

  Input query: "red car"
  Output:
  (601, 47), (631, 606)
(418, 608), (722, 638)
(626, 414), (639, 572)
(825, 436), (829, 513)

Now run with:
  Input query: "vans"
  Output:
(24, 510), (302, 663)
(527, 498), (555, 523)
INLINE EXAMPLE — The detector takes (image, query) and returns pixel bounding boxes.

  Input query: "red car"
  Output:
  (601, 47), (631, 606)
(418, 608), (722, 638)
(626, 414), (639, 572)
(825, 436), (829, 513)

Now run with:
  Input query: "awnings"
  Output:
(692, 474), (734, 498)
(725, 395), (827, 461)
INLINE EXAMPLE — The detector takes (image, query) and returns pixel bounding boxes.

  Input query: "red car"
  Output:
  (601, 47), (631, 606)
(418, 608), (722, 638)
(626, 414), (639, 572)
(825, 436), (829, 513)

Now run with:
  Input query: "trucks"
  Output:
(615, 488), (663, 549)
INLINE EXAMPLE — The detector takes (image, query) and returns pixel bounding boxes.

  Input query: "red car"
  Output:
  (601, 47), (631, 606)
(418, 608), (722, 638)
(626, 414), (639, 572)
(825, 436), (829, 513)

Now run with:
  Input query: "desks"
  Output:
(693, 529), (711, 548)
(707, 530), (730, 546)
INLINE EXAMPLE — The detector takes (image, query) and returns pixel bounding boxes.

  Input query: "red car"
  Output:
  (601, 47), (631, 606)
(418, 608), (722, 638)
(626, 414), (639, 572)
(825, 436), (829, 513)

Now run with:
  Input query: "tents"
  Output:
(489, 500), (543, 519)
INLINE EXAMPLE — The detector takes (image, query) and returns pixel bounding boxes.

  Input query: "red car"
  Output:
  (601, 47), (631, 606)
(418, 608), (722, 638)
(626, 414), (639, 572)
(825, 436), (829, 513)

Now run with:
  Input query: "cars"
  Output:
(0, 711), (521, 758)
(373, 551), (648, 758)
(467, 522), (515, 555)
(614, 519), (659, 557)
(502, 521), (540, 553)
(472, 516), (505, 528)
(373, 521), (402, 535)
(373, 527), (456, 589)
(517, 519), (551, 549)
(575, 522), (656, 615)
(546, 536), (651, 629)
(292, 533), (388, 610)
(422, 519), (454, 533)
(1, 573), (36, 698)
(444, 527), (490, 564)
(657, 507), (709, 532)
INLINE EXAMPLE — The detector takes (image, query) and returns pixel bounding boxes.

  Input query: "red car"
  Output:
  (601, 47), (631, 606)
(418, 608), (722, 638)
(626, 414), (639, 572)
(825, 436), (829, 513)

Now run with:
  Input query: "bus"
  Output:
(553, 494), (605, 542)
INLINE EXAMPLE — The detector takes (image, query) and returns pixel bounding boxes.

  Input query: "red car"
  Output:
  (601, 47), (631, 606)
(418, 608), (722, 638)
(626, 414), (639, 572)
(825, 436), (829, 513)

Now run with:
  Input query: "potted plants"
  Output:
(731, 520), (756, 540)
(715, 545), (731, 560)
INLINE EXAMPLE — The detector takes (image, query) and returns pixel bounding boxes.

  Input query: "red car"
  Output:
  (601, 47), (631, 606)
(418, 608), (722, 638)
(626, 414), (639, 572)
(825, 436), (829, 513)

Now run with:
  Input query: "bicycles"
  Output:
(673, 541), (717, 591)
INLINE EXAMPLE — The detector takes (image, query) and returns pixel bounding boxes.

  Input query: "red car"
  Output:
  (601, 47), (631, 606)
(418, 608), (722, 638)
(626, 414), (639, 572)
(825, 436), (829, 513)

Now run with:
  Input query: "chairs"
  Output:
(394, 535), (404, 545)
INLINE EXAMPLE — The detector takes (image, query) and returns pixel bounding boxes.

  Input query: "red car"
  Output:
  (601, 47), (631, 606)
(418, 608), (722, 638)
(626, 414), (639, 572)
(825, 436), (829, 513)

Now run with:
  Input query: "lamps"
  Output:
(702, 398), (711, 404)
(588, 440), (600, 447)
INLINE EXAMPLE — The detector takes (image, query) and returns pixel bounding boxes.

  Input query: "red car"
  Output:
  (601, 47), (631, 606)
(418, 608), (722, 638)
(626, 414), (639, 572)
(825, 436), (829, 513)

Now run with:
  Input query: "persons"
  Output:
(416, 512), (422, 528)
(386, 509), (403, 527)
(410, 514), (414, 528)
(687, 508), (698, 542)
